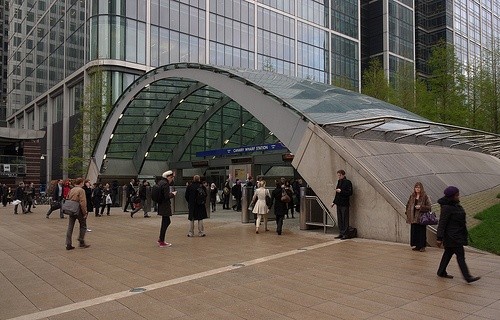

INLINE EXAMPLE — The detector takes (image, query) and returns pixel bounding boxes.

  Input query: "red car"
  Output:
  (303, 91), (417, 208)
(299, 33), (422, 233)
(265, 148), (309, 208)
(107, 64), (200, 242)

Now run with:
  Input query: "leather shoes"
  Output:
(466, 276), (480, 283)
(437, 272), (453, 279)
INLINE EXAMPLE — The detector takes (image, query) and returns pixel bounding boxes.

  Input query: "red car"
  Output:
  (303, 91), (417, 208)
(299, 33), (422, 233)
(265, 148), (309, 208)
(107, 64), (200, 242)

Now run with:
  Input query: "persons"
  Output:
(157, 170), (177, 247)
(203, 176), (305, 235)
(65, 178), (90, 250)
(405, 182), (431, 252)
(330, 170), (353, 240)
(185, 175), (208, 237)
(437, 186), (481, 283)
(0, 179), (158, 232)
(186, 180), (190, 187)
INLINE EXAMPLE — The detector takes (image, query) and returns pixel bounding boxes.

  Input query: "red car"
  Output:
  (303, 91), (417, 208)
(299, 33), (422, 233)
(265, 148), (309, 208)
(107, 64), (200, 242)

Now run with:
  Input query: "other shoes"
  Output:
(412, 247), (419, 250)
(335, 235), (341, 239)
(420, 247), (425, 252)
(79, 244), (91, 248)
(197, 232), (206, 236)
(341, 236), (349, 240)
(87, 229), (91, 232)
(66, 246), (75, 250)
(186, 232), (192, 237)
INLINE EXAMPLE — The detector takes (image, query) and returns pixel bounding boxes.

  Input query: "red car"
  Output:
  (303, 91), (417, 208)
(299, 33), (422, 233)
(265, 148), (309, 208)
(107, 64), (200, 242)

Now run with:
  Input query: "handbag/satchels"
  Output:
(197, 184), (206, 205)
(265, 190), (271, 206)
(349, 228), (356, 239)
(420, 205), (438, 226)
(62, 196), (80, 216)
(106, 194), (112, 204)
(51, 202), (61, 211)
(281, 189), (291, 203)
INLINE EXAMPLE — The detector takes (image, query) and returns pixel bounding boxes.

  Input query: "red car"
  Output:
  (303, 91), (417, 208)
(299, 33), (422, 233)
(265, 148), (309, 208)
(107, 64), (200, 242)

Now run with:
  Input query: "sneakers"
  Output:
(157, 240), (161, 244)
(160, 241), (171, 247)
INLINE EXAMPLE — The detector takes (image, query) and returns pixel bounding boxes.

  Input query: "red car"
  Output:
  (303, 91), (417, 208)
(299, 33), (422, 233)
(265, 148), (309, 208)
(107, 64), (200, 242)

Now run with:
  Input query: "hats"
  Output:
(444, 186), (459, 197)
(162, 170), (172, 179)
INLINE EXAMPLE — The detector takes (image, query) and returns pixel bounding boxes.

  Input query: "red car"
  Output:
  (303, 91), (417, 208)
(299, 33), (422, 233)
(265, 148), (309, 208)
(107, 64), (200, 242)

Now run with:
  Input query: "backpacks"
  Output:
(152, 186), (164, 202)
